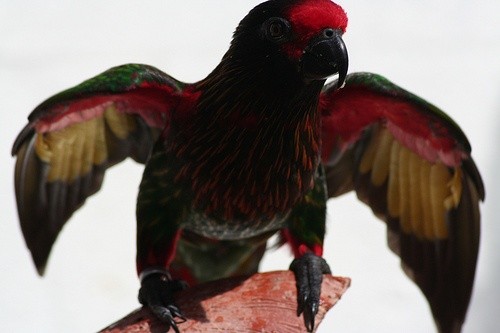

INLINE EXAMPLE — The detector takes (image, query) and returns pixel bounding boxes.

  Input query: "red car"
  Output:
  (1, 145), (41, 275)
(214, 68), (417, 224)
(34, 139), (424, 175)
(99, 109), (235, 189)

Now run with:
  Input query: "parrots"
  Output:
(12, 0), (488, 332)
